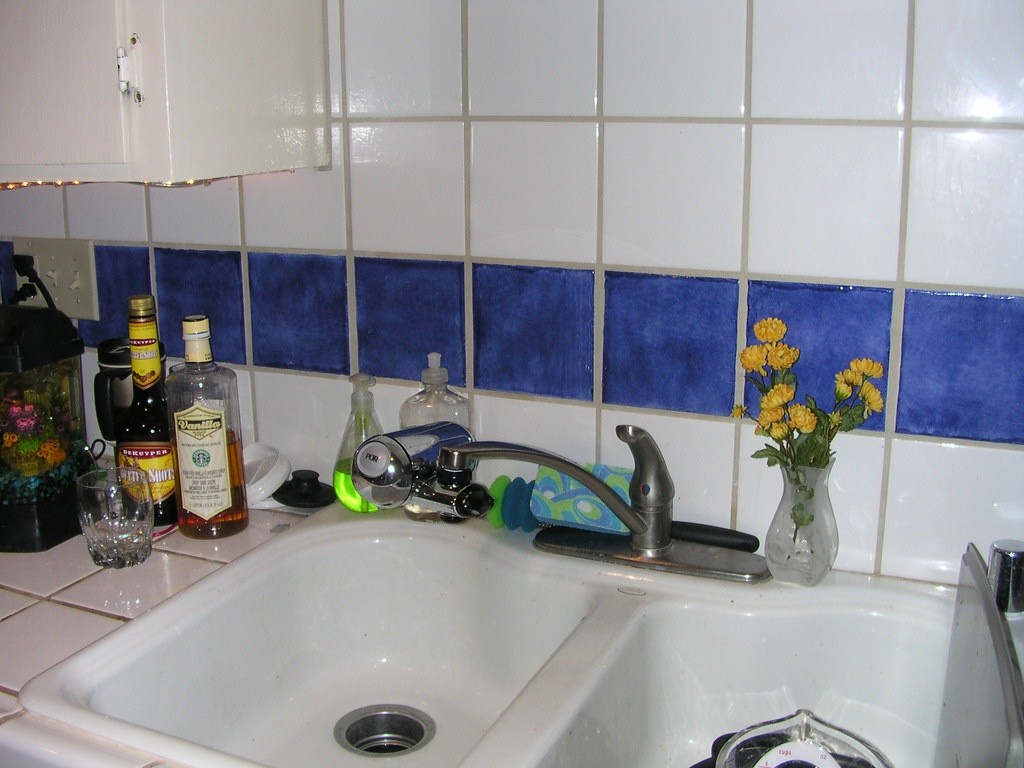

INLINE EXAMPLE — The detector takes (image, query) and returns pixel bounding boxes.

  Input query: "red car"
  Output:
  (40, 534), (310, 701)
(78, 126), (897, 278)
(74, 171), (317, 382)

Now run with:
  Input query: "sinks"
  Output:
(537, 609), (1011, 767)
(84, 524), (599, 768)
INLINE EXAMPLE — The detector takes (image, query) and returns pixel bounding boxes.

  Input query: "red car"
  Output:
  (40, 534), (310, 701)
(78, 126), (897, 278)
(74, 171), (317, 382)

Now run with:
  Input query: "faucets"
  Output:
(437, 423), (678, 555)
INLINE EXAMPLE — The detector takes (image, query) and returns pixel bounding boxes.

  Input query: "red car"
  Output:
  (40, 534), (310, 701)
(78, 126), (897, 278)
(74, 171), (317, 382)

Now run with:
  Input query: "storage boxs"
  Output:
(0, 305), (98, 553)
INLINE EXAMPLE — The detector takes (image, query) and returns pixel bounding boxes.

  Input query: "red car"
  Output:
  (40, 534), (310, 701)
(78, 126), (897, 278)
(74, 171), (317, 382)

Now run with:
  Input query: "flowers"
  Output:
(729, 317), (885, 544)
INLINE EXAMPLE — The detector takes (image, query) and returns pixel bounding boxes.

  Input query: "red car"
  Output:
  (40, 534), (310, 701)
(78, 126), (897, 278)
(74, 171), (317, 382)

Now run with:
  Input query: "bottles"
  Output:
(113, 295), (178, 527)
(165, 314), (250, 539)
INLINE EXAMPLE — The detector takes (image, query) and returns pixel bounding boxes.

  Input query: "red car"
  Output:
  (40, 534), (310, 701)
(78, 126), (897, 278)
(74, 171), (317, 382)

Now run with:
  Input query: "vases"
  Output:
(765, 457), (838, 586)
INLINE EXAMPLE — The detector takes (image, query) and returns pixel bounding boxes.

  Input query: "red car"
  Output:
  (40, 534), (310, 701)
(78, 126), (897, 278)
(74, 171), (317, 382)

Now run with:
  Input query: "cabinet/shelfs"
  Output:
(0, 1), (331, 188)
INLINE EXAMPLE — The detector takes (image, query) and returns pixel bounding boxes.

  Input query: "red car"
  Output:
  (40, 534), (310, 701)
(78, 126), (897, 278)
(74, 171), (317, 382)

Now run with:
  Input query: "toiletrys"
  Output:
(332, 371), (406, 514)
(398, 351), (471, 427)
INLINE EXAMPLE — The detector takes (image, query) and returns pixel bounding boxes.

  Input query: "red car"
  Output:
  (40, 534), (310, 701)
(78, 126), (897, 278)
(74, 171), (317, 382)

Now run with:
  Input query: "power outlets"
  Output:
(12, 236), (54, 307)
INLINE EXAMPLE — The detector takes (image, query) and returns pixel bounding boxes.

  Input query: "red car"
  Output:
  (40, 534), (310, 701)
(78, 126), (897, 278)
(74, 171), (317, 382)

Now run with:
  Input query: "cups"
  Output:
(93, 336), (171, 443)
(75, 467), (156, 568)
